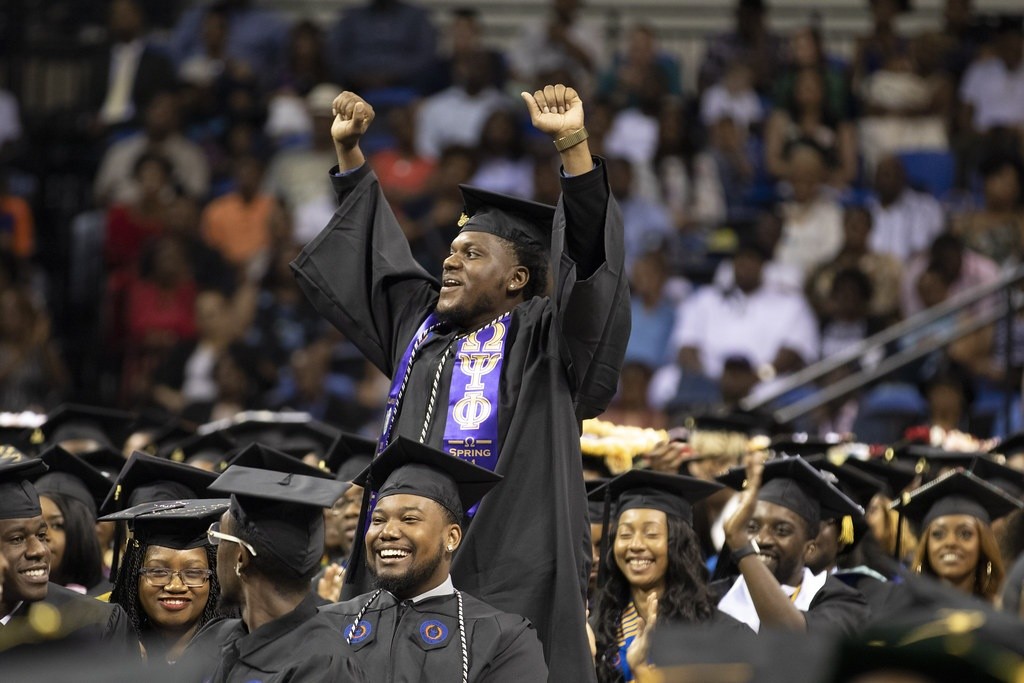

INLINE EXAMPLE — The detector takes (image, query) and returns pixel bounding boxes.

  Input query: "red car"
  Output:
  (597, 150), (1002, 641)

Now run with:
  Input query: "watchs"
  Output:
(730, 538), (760, 567)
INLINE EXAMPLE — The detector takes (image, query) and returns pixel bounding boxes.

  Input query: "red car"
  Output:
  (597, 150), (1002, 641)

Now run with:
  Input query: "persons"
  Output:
(0, 1), (1023, 683)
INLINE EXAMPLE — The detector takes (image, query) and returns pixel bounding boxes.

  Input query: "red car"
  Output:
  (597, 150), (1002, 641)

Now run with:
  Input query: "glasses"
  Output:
(136, 565), (214, 587)
(330, 492), (364, 515)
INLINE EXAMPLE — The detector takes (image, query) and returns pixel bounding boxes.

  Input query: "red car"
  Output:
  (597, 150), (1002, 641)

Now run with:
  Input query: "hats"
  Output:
(457, 182), (557, 257)
(341, 433), (505, 584)
(96, 499), (232, 583)
(583, 468), (727, 588)
(208, 463), (352, 577)
(715, 430), (1024, 546)
(0, 403), (378, 519)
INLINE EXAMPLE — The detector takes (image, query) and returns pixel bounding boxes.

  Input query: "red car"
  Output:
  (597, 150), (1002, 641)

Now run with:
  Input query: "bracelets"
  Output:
(553, 125), (589, 154)
